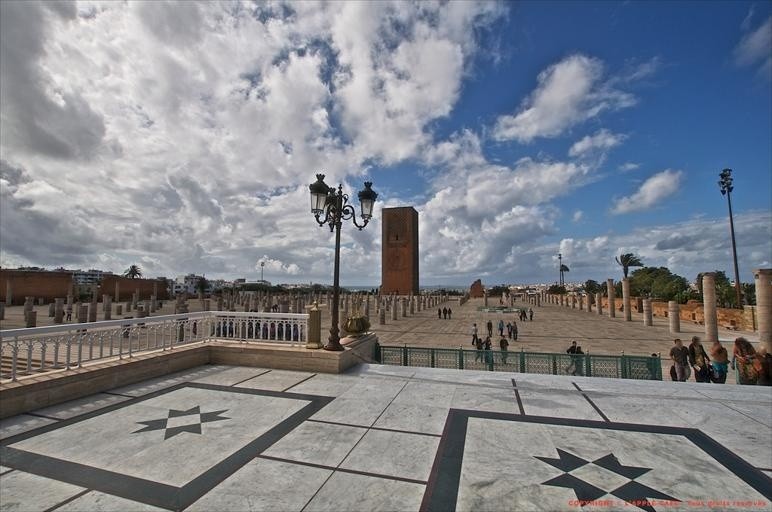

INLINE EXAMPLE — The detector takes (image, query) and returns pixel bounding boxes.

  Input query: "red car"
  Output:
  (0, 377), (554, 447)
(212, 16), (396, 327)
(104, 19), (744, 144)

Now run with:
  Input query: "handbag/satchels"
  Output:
(669, 361), (677, 381)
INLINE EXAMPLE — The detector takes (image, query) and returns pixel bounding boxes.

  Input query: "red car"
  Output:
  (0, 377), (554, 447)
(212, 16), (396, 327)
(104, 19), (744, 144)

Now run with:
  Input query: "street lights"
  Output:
(558, 253), (563, 286)
(717, 166), (744, 310)
(307, 171), (381, 352)
(260, 261), (265, 284)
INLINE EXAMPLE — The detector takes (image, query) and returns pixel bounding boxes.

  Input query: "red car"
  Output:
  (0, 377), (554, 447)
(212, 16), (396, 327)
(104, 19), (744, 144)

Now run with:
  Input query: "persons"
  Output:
(570, 344), (586, 377)
(649, 334), (772, 387)
(66, 308), (73, 321)
(566, 340), (578, 375)
(437, 304), (534, 365)
